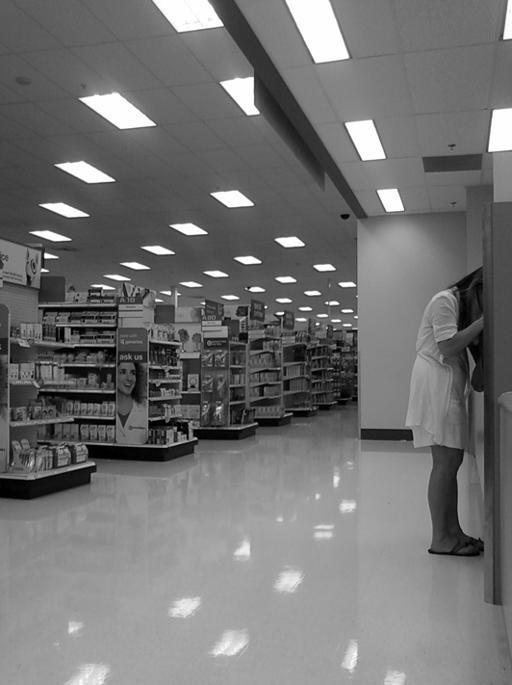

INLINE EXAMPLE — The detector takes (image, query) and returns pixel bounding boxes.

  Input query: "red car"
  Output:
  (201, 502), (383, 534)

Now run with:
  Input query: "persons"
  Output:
(116, 360), (148, 444)
(405, 266), (485, 556)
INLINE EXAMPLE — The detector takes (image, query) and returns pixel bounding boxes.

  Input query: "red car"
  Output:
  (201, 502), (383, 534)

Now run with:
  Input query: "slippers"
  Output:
(470, 538), (485, 553)
(428, 539), (481, 557)
